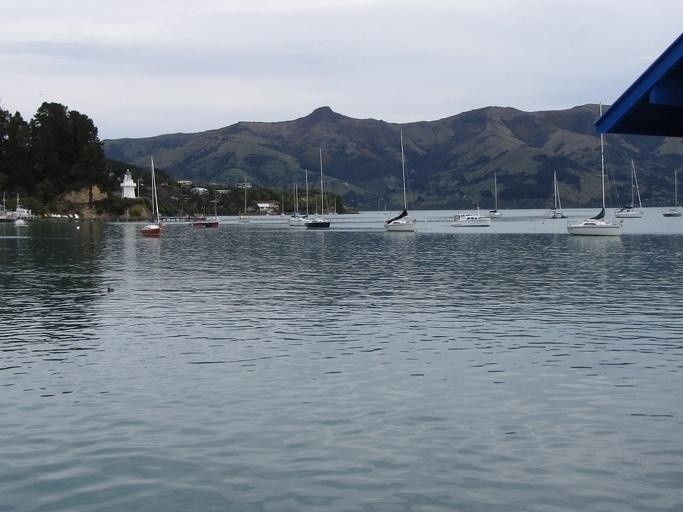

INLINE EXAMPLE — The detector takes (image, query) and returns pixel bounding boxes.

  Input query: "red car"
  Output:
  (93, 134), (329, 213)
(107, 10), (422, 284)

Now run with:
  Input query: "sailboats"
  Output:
(567, 99), (627, 237)
(287, 146), (333, 231)
(615, 154), (647, 221)
(383, 130), (417, 232)
(546, 171), (567, 219)
(485, 168), (502, 220)
(660, 166), (681, 220)
(237, 175), (251, 225)
(140, 154), (162, 238)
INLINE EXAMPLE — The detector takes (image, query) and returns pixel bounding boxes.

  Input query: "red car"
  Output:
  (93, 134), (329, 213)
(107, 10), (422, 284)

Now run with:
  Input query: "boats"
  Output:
(449, 214), (492, 229)
(0, 192), (39, 223)
(190, 219), (220, 229)
